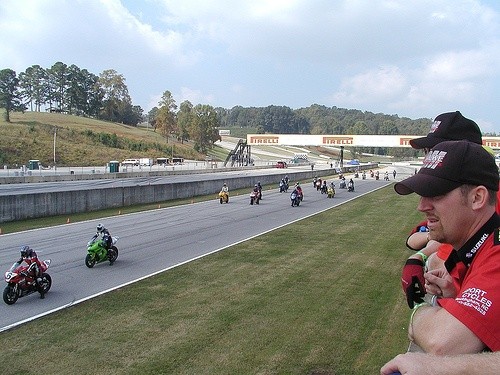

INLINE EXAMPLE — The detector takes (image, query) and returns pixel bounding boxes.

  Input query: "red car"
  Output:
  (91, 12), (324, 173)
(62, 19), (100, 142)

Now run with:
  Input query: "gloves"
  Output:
(400, 258), (427, 309)
(21, 272), (28, 276)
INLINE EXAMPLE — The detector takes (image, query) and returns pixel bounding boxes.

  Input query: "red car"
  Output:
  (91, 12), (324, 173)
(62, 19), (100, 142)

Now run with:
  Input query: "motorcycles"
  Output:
(2, 259), (52, 305)
(290, 184), (303, 207)
(250, 187), (262, 205)
(280, 182), (289, 192)
(313, 173), (389, 194)
(328, 186), (335, 198)
(219, 191), (229, 204)
(85, 235), (119, 268)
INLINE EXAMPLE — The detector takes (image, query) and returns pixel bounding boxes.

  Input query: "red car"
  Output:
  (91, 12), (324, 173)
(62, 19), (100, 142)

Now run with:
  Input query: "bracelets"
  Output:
(416, 251), (428, 263)
(411, 302), (431, 323)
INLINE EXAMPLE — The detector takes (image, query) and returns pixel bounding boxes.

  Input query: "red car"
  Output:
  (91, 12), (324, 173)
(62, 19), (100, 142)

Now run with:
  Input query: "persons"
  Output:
(9, 246), (46, 299)
(253, 181), (262, 204)
(220, 183), (229, 203)
(88, 224), (114, 266)
(381, 112), (500, 375)
(279, 163), (396, 206)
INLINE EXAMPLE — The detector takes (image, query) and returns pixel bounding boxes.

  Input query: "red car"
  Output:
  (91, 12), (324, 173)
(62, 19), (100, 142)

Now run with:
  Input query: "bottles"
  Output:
(419, 225), (428, 232)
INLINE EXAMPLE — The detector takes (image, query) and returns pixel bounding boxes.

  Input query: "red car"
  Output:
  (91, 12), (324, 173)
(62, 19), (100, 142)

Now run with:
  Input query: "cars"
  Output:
(277, 162), (288, 168)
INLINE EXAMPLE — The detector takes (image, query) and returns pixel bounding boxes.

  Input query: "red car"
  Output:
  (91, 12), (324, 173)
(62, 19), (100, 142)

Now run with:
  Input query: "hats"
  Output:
(394, 140), (500, 197)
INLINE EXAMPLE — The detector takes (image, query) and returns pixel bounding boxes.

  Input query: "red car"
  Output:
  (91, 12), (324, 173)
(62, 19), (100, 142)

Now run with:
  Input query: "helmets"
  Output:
(96, 224), (106, 232)
(410, 110), (482, 152)
(223, 183), (227, 187)
(21, 246), (30, 259)
(254, 184), (258, 189)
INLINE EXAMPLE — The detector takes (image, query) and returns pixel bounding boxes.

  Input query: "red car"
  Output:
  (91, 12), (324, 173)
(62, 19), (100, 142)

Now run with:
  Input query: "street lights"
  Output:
(53, 125), (59, 162)
(171, 130), (174, 165)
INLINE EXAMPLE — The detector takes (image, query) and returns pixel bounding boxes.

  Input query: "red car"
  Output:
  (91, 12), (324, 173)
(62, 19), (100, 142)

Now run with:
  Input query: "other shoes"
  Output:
(109, 262), (114, 265)
(40, 292), (44, 299)
